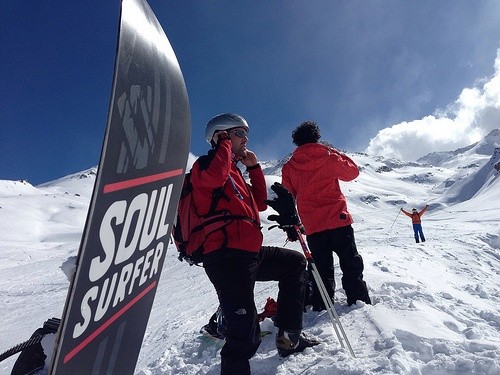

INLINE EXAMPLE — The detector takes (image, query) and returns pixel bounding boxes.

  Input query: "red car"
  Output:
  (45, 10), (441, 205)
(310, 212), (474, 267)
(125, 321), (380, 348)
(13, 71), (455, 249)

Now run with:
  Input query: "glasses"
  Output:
(228, 130), (249, 138)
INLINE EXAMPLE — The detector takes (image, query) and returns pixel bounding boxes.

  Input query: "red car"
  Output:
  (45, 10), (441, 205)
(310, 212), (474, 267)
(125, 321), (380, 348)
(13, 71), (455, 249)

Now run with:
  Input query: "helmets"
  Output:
(204, 112), (249, 146)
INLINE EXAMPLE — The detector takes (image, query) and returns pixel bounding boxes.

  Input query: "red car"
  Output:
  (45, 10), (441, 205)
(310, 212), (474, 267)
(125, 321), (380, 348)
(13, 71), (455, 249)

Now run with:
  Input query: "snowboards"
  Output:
(45, 0), (194, 375)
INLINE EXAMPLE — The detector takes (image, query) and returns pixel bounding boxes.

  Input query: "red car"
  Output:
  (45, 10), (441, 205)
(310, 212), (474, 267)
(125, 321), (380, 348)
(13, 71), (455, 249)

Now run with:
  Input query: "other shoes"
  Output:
(274, 332), (322, 358)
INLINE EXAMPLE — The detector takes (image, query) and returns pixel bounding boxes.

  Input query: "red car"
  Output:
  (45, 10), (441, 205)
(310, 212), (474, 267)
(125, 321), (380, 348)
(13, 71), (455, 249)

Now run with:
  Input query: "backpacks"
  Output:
(170, 154), (263, 267)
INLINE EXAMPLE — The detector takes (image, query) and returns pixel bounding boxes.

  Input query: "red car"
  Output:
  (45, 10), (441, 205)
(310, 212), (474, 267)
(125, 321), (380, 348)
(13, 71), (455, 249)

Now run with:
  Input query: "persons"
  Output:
(400, 204), (430, 243)
(282, 120), (371, 312)
(188, 113), (321, 375)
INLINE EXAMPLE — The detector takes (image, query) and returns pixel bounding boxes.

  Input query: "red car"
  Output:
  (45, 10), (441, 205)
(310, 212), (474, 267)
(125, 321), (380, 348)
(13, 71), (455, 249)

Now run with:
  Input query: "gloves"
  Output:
(263, 181), (305, 247)
